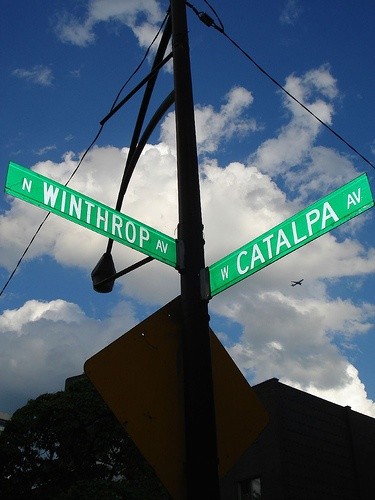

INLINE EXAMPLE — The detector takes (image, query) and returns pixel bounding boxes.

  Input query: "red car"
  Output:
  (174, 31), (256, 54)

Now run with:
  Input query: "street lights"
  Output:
(92, 36), (219, 499)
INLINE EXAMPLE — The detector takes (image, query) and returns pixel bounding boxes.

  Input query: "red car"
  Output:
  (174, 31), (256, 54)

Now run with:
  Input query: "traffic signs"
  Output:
(207, 171), (375, 298)
(4, 160), (179, 271)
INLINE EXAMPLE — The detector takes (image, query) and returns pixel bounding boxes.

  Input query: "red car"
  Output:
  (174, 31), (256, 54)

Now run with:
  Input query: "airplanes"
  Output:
(290, 279), (305, 287)
